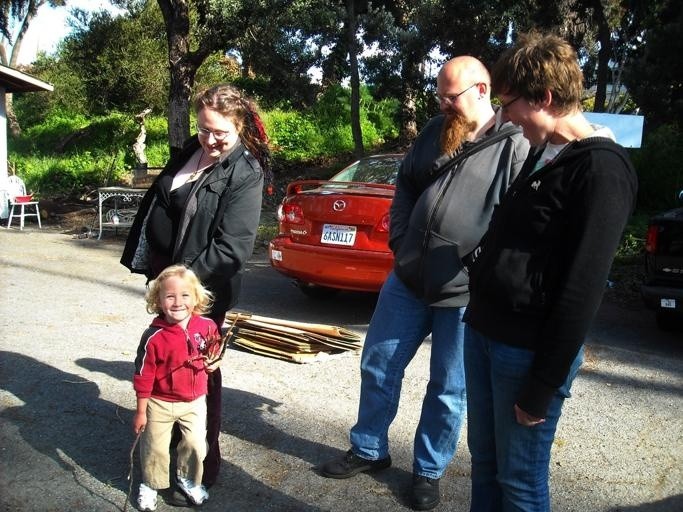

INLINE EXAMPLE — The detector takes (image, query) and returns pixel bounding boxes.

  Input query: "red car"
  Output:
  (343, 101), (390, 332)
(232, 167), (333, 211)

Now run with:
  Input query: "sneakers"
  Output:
(171, 482), (207, 507)
(137, 483), (158, 512)
(175, 473), (209, 505)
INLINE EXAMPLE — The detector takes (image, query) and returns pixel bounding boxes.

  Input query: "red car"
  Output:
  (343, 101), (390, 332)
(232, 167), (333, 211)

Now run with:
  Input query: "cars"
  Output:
(642, 191), (683, 330)
(267, 153), (405, 295)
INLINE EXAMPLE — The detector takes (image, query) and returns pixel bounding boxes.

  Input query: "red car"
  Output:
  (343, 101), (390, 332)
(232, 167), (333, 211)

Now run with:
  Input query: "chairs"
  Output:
(4, 175), (43, 232)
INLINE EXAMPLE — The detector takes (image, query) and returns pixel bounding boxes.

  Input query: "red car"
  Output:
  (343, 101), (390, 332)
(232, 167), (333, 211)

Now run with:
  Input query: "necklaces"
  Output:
(183, 150), (218, 182)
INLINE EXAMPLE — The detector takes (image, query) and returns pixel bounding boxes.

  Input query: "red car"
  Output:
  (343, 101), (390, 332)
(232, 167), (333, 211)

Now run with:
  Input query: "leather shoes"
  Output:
(411, 473), (440, 511)
(319, 448), (392, 478)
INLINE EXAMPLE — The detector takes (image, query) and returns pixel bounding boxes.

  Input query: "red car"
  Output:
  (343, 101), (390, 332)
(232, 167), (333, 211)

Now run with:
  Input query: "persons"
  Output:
(120, 84), (265, 491)
(133, 265), (225, 509)
(321, 54), (534, 511)
(462, 32), (637, 512)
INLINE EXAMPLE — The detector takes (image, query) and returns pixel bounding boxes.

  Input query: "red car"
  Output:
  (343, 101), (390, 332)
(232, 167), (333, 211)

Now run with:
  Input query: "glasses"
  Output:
(433, 83), (477, 104)
(500, 96), (522, 112)
(196, 123), (228, 141)
